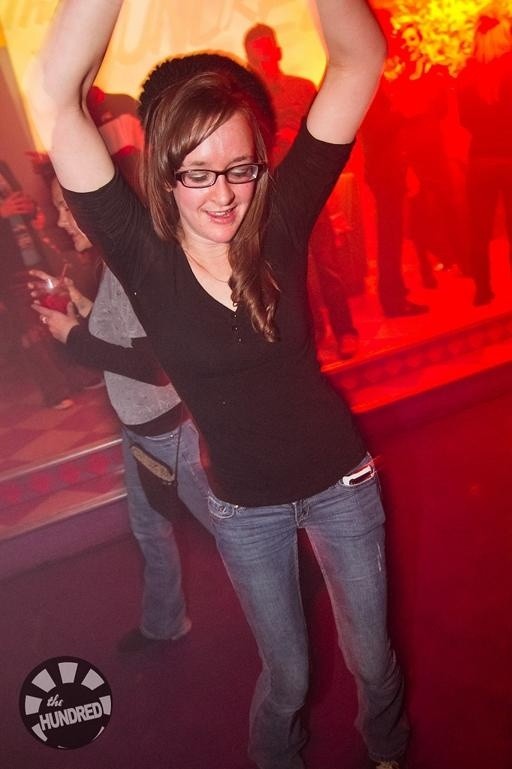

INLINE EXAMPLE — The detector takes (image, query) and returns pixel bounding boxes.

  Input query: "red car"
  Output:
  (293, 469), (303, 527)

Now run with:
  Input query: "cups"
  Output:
(35, 277), (72, 318)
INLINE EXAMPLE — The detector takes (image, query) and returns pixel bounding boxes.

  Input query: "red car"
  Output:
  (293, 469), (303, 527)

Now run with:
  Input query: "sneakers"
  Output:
(375, 760), (400, 769)
(118, 629), (190, 653)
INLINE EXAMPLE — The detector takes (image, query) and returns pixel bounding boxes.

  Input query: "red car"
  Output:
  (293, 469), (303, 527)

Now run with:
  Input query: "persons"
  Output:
(28, 1), (410, 769)
(1, 2), (511, 410)
(28, 177), (213, 655)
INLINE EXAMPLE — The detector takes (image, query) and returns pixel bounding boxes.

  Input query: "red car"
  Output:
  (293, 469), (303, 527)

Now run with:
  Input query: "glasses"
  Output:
(172, 163), (261, 189)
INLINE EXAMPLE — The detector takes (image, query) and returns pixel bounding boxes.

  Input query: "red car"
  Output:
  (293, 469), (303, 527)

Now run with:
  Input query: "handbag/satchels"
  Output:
(127, 442), (177, 504)
